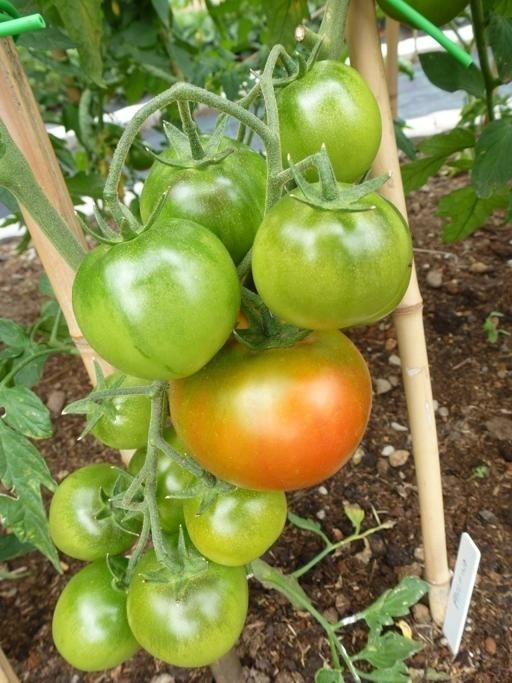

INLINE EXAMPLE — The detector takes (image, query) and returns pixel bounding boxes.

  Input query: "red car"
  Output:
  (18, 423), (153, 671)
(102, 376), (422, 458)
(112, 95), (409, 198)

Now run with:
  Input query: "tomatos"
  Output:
(48, 56), (413, 670)
